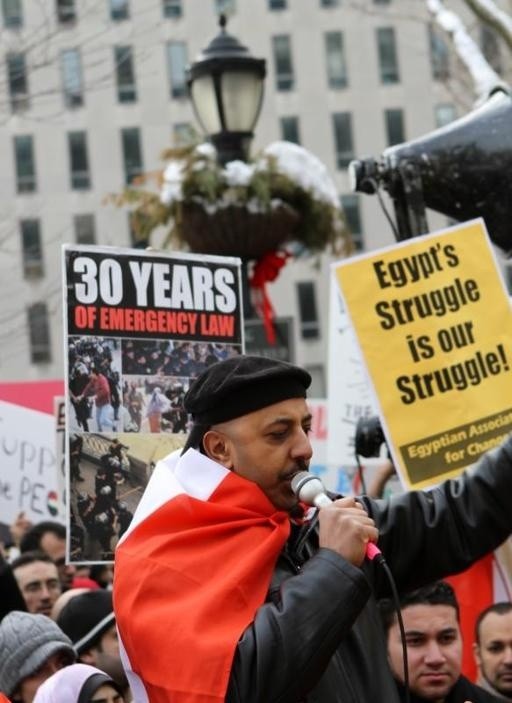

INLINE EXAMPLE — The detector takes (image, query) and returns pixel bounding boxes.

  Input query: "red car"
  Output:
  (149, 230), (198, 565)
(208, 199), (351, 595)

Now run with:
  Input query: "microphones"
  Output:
(289, 471), (385, 567)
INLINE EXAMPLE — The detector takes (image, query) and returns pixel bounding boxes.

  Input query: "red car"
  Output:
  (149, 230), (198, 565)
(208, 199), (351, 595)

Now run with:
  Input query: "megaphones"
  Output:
(350, 86), (512, 256)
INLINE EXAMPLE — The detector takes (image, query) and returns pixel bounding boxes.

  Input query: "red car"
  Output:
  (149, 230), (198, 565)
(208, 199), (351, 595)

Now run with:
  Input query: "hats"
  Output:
(56, 590), (118, 653)
(183, 356), (311, 458)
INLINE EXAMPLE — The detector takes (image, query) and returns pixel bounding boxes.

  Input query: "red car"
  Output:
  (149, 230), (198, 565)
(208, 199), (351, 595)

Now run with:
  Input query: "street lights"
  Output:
(185, 13), (269, 320)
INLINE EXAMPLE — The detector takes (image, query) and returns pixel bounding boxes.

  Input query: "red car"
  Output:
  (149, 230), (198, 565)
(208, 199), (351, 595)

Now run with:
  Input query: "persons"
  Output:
(383, 579), (503, 702)
(122, 338), (244, 377)
(32, 663), (126, 703)
(470, 601), (510, 702)
(114, 358), (511, 703)
(11, 553), (60, 618)
(72, 438), (132, 562)
(21, 517), (77, 596)
(122, 376), (190, 433)
(68, 332), (121, 431)
(57, 591), (130, 685)
